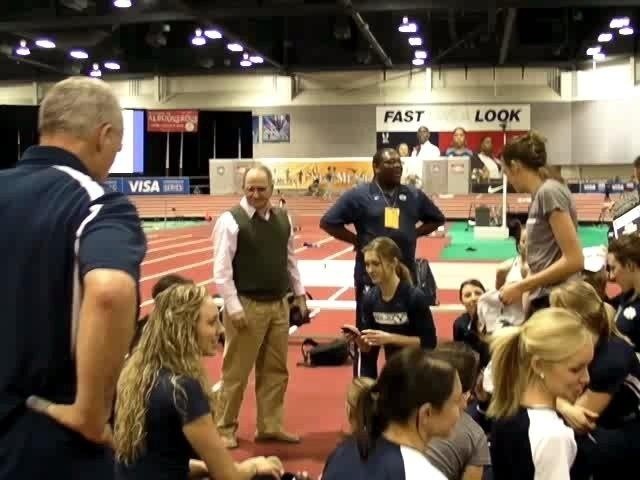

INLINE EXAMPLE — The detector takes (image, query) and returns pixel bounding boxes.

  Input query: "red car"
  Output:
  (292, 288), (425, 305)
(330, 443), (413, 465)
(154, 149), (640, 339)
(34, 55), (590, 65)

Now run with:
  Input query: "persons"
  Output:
(410, 126), (441, 158)
(470, 134), (504, 195)
(319, 145), (446, 403)
(115, 284), (297, 480)
(212, 163), (309, 449)
(444, 127), (491, 193)
(606, 154), (640, 248)
(0, 72), (149, 480)
(496, 131), (585, 329)
(321, 218), (640, 480)
(129, 274), (198, 353)
(395, 142), (413, 158)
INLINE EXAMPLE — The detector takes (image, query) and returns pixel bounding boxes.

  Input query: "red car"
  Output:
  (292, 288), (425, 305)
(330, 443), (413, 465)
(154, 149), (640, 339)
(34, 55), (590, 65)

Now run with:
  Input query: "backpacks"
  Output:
(416, 259), (436, 305)
(301, 336), (357, 366)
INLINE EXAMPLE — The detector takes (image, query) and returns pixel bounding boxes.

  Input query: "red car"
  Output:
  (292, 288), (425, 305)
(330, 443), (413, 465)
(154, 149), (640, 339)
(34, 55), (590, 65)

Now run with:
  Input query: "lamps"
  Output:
(192, 28), (206, 45)
(90, 63), (101, 77)
(240, 52), (252, 67)
(16, 39), (30, 56)
(398, 16), (410, 33)
